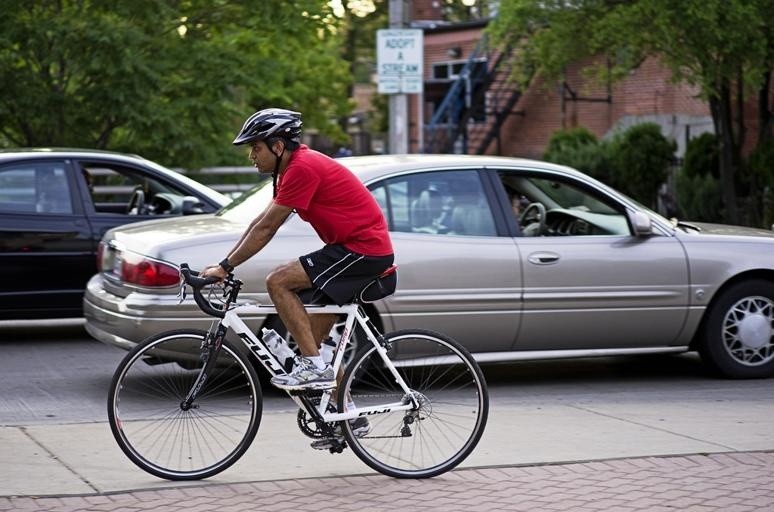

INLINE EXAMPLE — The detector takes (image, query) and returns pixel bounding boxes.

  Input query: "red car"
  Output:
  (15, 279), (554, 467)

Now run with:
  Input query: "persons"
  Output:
(196, 106), (395, 448)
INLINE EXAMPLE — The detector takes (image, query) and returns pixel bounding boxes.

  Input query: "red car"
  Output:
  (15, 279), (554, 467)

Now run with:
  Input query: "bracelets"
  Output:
(219, 258), (234, 272)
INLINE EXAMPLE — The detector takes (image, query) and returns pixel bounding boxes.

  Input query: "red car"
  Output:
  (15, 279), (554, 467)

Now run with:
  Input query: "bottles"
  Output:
(262, 326), (299, 368)
(319, 336), (337, 366)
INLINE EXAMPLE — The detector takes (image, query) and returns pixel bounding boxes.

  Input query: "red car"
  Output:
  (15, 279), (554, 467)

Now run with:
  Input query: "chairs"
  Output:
(419, 190), (492, 234)
(37, 175), (61, 213)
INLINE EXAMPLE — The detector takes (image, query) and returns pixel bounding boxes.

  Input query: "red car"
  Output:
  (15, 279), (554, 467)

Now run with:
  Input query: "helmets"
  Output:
(232, 108), (303, 145)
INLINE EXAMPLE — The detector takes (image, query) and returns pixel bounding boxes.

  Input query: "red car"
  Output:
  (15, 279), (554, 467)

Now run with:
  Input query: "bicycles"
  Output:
(106, 267), (489, 478)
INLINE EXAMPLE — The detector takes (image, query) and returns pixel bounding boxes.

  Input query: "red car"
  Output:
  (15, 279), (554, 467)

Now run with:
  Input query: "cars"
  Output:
(0, 146), (237, 322)
(82, 154), (774, 379)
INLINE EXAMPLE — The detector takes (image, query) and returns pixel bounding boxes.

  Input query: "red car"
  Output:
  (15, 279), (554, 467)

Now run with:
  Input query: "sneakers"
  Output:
(311, 417), (370, 450)
(270, 357), (337, 390)
